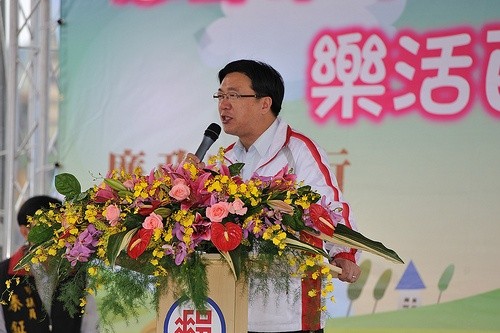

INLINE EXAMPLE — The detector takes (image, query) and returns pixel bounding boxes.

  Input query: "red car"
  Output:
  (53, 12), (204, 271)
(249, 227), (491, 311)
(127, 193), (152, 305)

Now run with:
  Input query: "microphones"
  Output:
(195, 123), (221, 163)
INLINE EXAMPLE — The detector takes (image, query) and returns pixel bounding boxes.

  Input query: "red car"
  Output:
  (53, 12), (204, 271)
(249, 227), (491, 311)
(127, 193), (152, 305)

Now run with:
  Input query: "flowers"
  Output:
(0, 147), (405, 333)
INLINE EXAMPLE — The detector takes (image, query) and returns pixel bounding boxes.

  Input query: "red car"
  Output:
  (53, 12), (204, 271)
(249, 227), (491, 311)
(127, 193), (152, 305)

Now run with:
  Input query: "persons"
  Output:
(0, 196), (83, 333)
(177, 58), (361, 333)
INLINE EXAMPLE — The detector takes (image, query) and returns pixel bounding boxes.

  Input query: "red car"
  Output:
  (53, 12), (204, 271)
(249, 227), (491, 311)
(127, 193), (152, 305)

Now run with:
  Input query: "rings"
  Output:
(352, 275), (357, 278)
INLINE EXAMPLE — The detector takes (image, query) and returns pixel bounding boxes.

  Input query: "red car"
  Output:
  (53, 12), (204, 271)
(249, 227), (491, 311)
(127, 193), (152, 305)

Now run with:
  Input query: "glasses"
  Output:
(213, 91), (260, 102)
